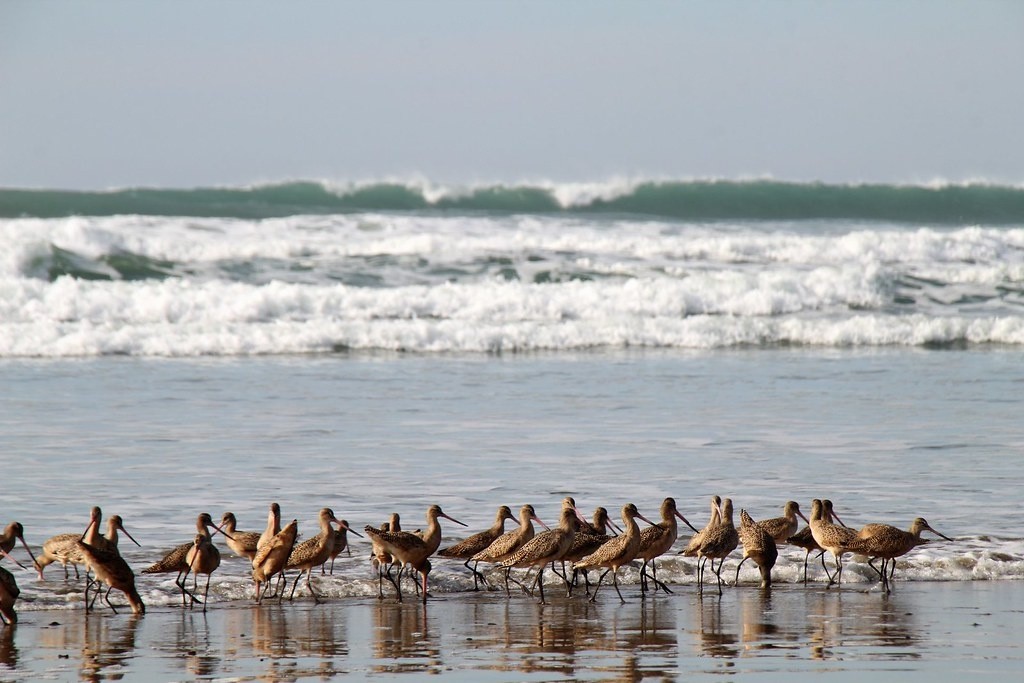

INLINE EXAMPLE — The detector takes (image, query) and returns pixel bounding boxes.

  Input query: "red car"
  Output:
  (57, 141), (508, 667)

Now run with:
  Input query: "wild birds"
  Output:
(438, 496), (951, 607)
(141, 502), (469, 605)
(0, 507), (146, 626)
